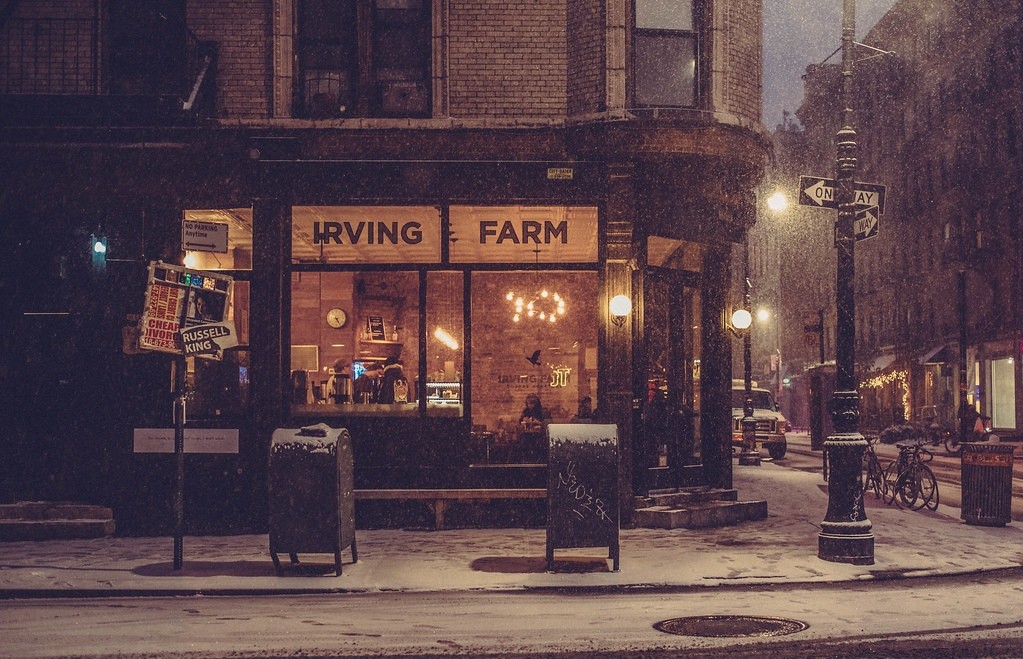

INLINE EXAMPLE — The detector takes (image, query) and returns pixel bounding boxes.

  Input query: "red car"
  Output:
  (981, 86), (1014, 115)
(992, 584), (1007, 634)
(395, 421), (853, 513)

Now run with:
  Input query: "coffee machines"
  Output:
(331, 373), (352, 405)
(290, 366), (315, 405)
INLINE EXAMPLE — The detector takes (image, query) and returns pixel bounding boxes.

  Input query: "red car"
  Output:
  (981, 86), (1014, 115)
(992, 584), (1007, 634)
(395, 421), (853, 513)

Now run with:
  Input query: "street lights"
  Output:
(734, 192), (788, 466)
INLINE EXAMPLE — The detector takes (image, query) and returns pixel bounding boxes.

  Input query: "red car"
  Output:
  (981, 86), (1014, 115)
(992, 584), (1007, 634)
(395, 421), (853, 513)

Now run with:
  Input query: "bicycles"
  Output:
(861, 427), (994, 511)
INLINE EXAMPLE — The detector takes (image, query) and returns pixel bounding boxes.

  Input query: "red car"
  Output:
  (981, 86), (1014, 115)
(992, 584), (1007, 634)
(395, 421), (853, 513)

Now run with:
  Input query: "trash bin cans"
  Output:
(957, 440), (1018, 526)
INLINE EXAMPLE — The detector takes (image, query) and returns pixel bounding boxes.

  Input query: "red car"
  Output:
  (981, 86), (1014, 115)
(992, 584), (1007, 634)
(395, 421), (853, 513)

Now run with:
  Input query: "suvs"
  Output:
(732, 379), (788, 462)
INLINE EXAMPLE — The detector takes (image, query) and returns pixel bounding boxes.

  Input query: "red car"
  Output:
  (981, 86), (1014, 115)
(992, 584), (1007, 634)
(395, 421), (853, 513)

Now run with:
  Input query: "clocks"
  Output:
(325, 307), (347, 328)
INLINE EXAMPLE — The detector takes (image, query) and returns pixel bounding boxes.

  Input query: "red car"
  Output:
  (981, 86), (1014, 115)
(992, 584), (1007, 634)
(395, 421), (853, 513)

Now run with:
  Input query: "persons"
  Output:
(354, 361), (384, 405)
(326, 360), (352, 404)
(578, 396), (593, 419)
(518, 392), (553, 428)
(377, 356), (411, 404)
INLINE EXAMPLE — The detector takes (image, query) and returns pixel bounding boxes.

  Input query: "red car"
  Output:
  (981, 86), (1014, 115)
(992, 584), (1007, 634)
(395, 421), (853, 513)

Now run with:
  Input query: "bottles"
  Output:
(391, 324), (399, 342)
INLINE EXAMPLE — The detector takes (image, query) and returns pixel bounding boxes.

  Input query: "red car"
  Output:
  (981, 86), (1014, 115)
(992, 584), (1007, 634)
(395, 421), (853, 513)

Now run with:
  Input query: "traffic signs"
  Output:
(833, 205), (879, 249)
(798, 176), (887, 216)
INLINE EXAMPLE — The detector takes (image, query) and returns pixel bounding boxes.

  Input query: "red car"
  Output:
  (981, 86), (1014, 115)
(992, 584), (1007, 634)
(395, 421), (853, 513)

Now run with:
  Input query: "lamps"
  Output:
(503, 273), (567, 325)
